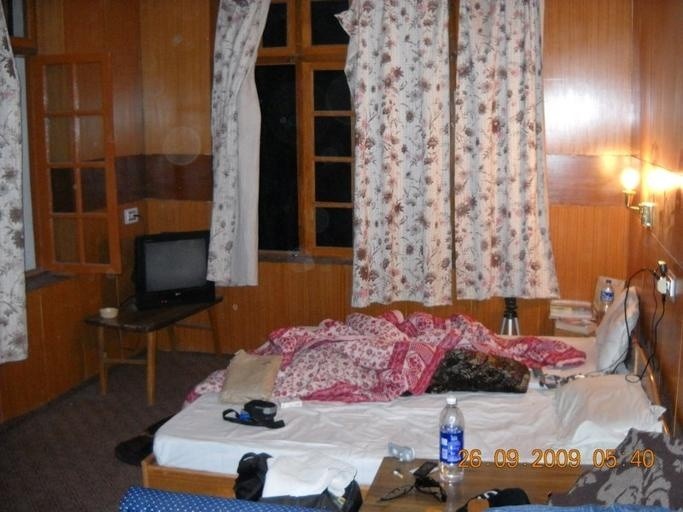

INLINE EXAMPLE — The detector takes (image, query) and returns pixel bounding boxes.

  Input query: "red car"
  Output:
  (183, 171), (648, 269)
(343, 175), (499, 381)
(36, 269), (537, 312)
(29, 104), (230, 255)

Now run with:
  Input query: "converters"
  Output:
(656, 259), (666, 274)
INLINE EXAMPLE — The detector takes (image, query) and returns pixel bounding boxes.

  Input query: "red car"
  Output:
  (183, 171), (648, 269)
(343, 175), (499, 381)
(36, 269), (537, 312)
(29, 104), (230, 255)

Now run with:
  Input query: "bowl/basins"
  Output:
(99, 307), (119, 319)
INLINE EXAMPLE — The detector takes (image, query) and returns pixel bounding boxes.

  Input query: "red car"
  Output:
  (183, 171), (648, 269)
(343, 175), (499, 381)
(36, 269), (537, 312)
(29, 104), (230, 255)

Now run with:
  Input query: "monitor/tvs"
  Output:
(134, 231), (216, 309)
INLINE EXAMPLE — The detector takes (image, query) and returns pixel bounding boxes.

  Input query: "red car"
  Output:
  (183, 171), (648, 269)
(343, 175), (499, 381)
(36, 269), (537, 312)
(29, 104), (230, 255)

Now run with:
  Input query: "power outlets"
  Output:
(125, 208), (139, 223)
(655, 274), (675, 299)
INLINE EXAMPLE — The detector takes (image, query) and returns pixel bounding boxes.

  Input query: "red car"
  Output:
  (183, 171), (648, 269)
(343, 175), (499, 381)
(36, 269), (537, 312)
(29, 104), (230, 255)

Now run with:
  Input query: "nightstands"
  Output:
(549, 309), (607, 338)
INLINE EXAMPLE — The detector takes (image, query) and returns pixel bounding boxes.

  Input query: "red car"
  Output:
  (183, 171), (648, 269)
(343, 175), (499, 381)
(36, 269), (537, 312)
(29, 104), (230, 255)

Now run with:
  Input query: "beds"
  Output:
(141, 324), (670, 495)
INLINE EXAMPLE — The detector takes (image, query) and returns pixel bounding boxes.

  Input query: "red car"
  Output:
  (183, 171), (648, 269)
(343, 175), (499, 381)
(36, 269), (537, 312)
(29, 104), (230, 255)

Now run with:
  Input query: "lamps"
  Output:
(616, 166), (656, 229)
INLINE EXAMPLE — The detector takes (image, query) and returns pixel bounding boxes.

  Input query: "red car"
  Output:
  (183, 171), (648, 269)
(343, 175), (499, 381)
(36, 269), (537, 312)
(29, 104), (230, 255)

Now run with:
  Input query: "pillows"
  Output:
(548, 428), (683, 509)
(596, 288), (639, 369)
(554, 376), (665, 450)
(220, 349), (281, 402)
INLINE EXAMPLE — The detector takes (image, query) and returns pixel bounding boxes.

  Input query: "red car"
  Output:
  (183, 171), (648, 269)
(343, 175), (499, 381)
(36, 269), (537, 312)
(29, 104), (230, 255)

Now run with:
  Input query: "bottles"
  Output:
(599, 278), (615, 324)
(436, 396), (465, 483)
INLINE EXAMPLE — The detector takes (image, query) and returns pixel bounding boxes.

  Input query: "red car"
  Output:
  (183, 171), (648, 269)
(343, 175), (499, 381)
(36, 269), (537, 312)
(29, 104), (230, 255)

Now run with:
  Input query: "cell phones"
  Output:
(413, 461), (435, 477)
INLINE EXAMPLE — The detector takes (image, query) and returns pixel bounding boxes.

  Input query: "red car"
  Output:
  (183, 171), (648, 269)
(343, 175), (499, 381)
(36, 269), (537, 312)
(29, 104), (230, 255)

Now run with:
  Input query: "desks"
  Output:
(358, 456), (683, 512)
(83, 295), (225, 407)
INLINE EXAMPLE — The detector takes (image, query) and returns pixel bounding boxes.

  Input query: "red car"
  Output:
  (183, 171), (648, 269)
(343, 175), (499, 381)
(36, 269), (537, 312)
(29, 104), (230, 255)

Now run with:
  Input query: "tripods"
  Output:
(499, 298), (519, 336)
